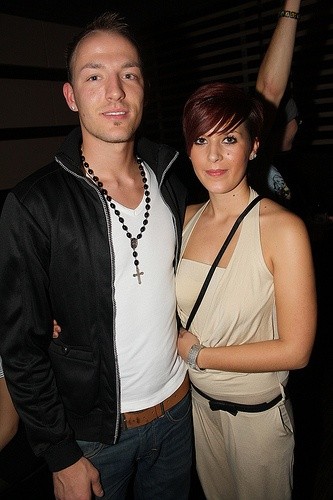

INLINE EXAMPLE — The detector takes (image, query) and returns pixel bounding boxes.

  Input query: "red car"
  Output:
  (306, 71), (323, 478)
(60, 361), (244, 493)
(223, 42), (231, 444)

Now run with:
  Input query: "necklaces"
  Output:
(80, 149), (151, 284)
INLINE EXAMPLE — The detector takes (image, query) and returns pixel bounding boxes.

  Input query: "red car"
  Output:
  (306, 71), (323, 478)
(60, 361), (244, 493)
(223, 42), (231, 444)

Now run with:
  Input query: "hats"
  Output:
(284, 98), (298, 122)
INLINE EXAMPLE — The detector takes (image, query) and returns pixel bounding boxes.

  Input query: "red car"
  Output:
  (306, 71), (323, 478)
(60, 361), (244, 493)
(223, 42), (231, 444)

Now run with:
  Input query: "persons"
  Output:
(0, 12), (205, 500)
(245, 0), (301, 210)
(175, 84), (316, 499)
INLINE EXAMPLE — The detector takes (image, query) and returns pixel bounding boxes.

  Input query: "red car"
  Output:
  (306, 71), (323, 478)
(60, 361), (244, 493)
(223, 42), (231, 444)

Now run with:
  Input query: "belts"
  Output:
(118, 372), (189, 430)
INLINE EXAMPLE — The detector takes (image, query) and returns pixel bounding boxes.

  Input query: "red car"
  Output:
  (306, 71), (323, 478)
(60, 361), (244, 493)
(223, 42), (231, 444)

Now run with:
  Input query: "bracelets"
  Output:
(280, 9), (299, 20)
(187, 343), (206, 373)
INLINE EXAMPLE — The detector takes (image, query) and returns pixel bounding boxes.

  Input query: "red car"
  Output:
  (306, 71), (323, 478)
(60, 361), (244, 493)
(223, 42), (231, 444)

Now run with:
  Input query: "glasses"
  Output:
(293, 118), (302, 125)
